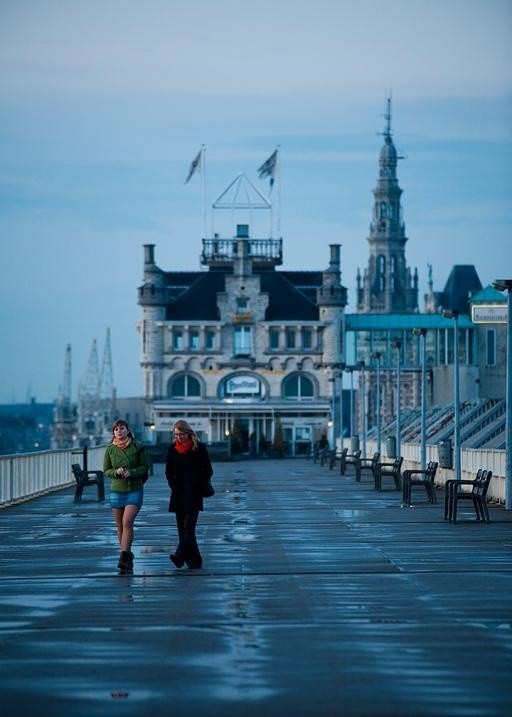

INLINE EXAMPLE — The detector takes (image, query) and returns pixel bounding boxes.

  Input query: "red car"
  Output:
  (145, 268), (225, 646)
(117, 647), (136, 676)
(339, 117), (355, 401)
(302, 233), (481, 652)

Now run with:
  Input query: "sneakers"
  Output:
(169, 554), (202, 569)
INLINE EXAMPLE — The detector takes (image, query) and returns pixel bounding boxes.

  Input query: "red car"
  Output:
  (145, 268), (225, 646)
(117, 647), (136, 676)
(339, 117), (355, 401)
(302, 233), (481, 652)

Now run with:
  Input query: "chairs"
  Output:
(317, 446), (494, 522)
(70, 463), (106, 502)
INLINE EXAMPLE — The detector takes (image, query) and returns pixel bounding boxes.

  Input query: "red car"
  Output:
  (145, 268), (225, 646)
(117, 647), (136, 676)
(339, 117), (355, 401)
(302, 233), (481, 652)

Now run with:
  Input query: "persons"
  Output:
(163, 419), (217, 570)
(110, 580), (135, 702)
(103, 417), (151, 571)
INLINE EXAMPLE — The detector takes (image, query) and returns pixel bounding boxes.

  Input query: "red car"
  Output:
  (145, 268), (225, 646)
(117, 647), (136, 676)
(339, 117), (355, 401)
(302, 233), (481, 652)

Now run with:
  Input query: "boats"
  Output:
(0, 323), (119, 455)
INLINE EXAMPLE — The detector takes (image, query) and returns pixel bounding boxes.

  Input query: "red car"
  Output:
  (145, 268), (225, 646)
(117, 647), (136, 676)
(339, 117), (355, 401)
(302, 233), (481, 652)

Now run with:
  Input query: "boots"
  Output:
(117, 551), (134, 574)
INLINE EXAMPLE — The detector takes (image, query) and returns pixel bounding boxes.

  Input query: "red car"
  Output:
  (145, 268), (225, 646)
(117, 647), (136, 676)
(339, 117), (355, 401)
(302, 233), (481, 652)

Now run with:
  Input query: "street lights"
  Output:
(491, 275), (512, 513)
(326, 306), (462, 492)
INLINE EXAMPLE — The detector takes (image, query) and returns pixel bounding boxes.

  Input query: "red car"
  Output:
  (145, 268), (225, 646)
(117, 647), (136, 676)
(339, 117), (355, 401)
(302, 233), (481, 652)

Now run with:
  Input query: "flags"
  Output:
(255, 149), (278, 179)
(183, 149), (203, 182)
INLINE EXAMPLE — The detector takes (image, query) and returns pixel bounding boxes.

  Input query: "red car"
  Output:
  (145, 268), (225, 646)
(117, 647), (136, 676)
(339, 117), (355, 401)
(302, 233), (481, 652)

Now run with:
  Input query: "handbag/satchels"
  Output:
(204, 480), (215, 497)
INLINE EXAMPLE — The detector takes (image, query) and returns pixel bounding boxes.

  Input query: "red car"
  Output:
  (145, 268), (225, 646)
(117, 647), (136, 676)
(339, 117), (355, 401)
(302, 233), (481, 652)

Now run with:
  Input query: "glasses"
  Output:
(174, 433), (185, 438)
